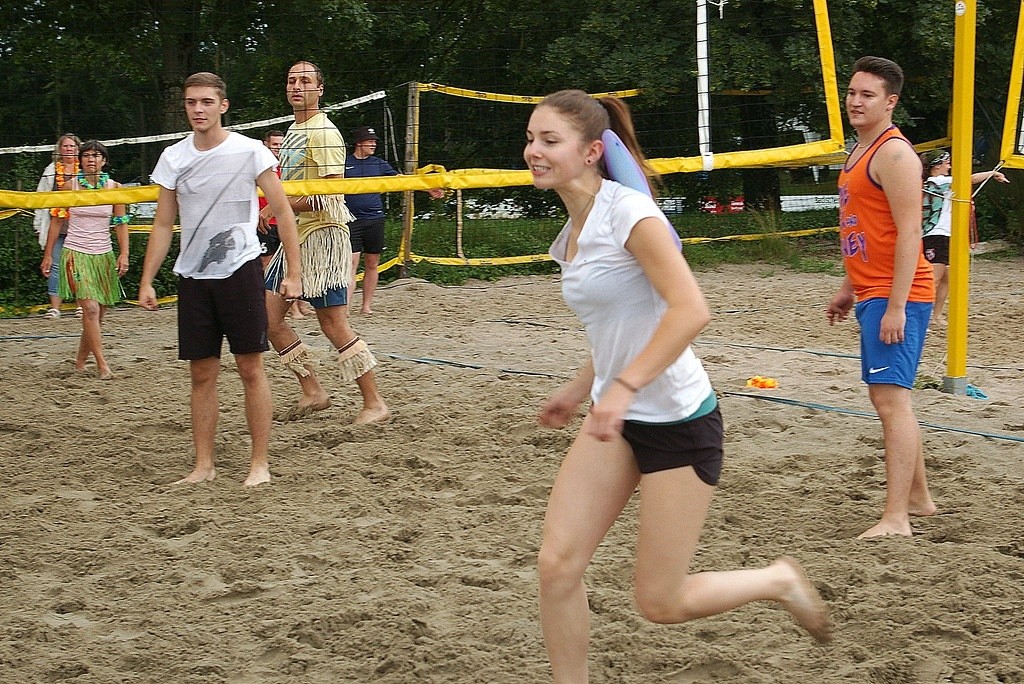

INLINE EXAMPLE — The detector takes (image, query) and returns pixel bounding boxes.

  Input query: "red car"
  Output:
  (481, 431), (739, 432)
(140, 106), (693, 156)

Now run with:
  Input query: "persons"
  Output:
(33, 133), (86, 319)
(342, 126), (446, 313)
(256, 129), (307, 323)
(523, 90), (833, 684)
(137, 72), (304, 488)
(40, 140), (133, 381)
(826, 54), (936, 538)
(261, 60), (392, 429)
(921, 148), (1012, 327)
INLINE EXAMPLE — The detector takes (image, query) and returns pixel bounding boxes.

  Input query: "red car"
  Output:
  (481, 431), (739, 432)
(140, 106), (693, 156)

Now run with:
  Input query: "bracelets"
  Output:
(615, 377), (638, 391)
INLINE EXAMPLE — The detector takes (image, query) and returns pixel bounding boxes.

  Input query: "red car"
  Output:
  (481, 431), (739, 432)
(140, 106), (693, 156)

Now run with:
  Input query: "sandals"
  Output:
(43, 309), (61, 318)
(74, 307), (84, 318)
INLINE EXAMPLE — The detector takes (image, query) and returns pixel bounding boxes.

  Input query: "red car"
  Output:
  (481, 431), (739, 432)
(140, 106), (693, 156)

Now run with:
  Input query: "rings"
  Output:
(125, 269), (128, 270)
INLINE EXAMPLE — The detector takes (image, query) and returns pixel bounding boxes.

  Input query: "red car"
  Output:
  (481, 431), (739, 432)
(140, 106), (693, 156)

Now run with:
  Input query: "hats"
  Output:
(353, 127), (379, 144)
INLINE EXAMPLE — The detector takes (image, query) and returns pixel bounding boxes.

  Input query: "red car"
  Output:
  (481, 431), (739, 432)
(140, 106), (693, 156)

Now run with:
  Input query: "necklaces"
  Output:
(56, 159), (80, 190)
(78, 171), (110, 190)
